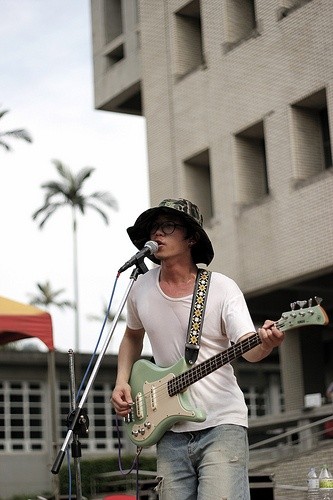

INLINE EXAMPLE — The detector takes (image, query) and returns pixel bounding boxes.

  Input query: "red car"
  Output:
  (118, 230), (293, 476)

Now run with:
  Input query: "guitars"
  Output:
(122, 296), (329, 446)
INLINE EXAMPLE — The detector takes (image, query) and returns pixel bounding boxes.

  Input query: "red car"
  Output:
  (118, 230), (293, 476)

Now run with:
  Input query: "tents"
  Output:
(0, 295), (54, 351)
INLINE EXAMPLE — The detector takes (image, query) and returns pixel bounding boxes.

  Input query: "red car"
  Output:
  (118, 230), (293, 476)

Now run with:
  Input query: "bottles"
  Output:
(319, 465), (333, 500)
(306, 468), (319, 500)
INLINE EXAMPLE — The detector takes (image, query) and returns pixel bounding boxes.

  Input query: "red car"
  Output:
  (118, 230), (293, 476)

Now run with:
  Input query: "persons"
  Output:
(110, 198), (285, 500)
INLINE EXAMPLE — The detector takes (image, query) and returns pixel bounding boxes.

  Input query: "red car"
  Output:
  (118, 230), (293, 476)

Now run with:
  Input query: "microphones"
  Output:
(118, 241), (159, 274)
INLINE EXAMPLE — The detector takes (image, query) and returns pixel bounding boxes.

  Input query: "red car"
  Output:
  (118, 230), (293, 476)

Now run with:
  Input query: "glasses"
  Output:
(147, 221), (187, 235)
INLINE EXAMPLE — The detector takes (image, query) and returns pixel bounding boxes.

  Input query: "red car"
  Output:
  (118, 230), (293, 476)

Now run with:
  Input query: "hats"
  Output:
(126, 197), (214, 267)
